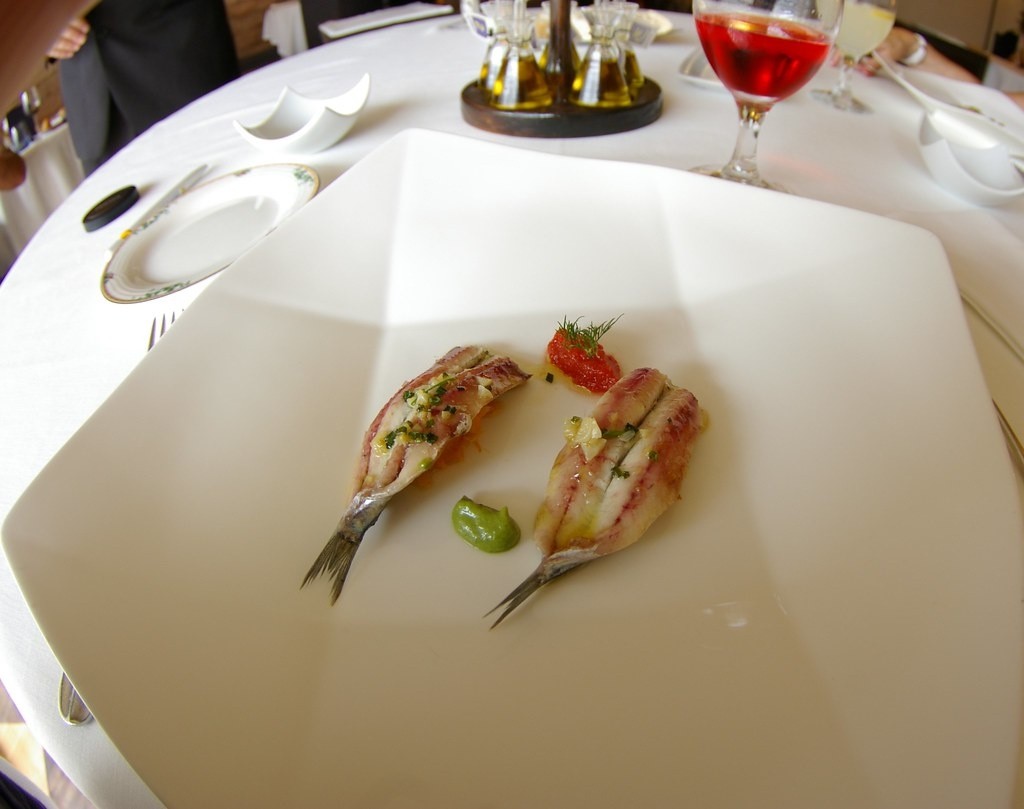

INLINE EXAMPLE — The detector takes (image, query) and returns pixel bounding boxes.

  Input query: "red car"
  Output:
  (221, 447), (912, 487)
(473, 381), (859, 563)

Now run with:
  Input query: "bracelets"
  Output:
(899, 33), (927, 67)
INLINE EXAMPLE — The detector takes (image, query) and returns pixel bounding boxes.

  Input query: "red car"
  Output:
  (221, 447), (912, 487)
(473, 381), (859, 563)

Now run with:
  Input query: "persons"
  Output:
(0, 0), (243, 193)
(831, 28), (1023, 111)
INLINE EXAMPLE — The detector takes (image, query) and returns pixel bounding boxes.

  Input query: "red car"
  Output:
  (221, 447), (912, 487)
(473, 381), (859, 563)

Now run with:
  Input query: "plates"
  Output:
(583, 6), (673, 41)
(916, 112), (1024, 206)
(99, 164), (320, 304)
(871, 50), (1024, 172)
(232, 73), (369, 155)
(2, 128), (1024, 809)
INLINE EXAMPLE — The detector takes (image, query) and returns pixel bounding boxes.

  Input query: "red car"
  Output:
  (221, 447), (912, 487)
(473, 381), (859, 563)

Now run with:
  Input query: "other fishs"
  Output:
(297, 339), (531, 602)
(476, 360), (711, 634)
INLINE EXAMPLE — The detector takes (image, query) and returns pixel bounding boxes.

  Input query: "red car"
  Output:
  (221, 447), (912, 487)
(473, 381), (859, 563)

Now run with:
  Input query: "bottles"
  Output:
(480, 1), (644, 107)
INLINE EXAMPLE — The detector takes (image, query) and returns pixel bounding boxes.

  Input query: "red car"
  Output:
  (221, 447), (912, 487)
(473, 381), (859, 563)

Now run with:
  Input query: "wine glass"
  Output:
(21, 86), (48, 139)
(688, 0), (844, 194)
(811, 0), (898, 116)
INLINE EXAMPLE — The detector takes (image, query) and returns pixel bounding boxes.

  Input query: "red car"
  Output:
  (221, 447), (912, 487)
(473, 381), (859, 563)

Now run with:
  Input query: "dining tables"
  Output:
(0, 0), (1024, 809)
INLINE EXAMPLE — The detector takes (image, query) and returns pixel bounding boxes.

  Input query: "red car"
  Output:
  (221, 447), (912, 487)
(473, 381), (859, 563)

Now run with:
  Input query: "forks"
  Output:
(148, 308), (184, 349)
